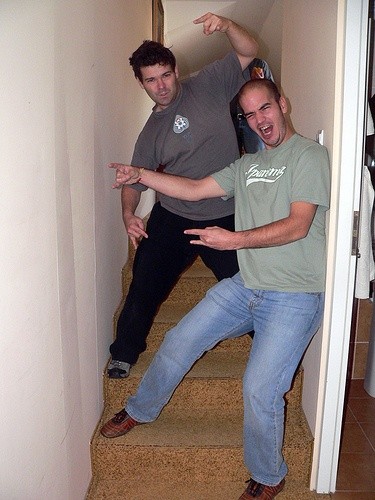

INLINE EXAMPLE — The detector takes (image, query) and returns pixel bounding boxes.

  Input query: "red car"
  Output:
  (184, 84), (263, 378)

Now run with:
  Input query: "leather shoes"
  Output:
(236, 476), (285, 500)
(99, 408), (145, 438)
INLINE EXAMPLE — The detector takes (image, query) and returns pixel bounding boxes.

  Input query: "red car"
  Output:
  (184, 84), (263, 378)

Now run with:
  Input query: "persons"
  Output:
(99, 77), (333, 499)
(104, 10), (261, 381)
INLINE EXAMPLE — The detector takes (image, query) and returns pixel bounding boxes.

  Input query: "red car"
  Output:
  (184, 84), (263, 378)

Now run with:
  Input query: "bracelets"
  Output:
(138, 167), (144, 182)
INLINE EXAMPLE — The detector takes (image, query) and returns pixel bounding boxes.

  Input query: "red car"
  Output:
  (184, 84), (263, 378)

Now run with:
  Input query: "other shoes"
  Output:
(107, 360), (131, 378)
